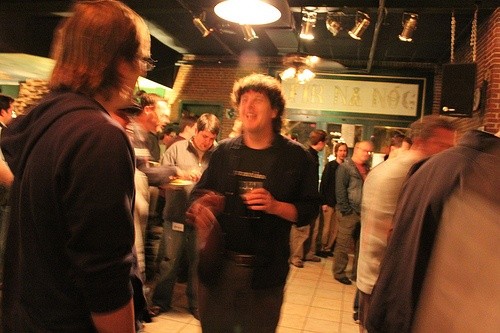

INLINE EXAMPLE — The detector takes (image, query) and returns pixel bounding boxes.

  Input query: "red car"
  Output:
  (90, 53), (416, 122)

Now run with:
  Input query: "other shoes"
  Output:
(190, 307), (200, 320)
(336, 276), (352, 284)
(148, 305), (161, 316)
(316, 249), (333, 258)
(292, 261), (303, 267)
(304, 256), (321, 262)
(353, 311), (360, 324)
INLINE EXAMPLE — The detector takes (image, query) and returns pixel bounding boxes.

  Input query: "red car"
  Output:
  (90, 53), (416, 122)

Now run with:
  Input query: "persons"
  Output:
(0, 90), (221, 318)
(0, 0), (155, 333)
(186, 74), (321, 333)
(353, 115), (500, 333)
(289, 128), (412, 285)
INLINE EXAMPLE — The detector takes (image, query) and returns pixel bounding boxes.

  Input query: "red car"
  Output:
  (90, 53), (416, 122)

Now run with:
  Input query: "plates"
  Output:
(168, 179), (196, 186)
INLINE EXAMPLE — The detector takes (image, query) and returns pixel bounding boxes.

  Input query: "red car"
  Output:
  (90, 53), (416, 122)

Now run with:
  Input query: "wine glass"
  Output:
(239, 180), (263, 220)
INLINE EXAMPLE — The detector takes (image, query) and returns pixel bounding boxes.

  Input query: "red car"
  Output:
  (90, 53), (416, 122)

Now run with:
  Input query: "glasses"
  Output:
(135, 53), (158, 71)
(367, 151), (375, 156)
(340, 150), (347, 153)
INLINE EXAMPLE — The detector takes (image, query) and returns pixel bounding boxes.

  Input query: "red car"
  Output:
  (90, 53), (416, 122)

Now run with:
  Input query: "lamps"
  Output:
(347, 10), (371, 41)
(325, 15), (344, 37)
(278, 52), (320, 84)
(398, 12), (418, 42)
(192, 0), (283, 42)
(299, 7), (318, 41)
